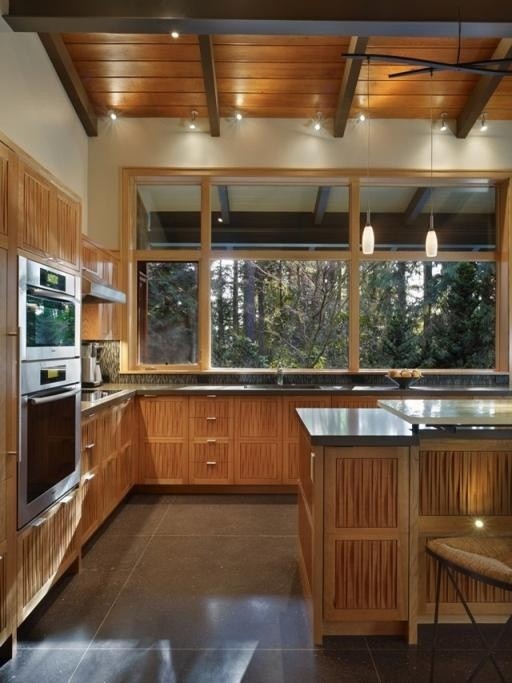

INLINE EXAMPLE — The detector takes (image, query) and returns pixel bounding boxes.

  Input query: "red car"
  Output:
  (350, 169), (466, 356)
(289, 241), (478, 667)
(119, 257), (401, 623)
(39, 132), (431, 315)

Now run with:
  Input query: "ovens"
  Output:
(19, 256), (83, 534)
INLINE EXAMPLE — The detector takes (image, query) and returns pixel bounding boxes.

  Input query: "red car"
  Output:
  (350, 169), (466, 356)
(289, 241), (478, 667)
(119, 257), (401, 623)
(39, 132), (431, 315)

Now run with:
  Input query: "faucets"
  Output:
(277, 361), (285, 385)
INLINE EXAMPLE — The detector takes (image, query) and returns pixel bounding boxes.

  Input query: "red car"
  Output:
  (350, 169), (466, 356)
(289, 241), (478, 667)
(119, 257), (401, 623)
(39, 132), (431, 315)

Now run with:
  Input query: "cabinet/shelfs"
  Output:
(2, 138), (125, 346)
(17, 488), (84, 632)
(80, 392), (140, 548)
(3, 541), (40, 658)
(135, 396), (377, 490)
(318, 435), (410, 655)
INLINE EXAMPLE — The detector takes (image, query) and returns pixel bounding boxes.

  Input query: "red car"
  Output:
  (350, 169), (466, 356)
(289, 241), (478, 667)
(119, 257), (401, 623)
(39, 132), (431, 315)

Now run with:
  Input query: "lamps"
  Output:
(342, 1), (512, 258)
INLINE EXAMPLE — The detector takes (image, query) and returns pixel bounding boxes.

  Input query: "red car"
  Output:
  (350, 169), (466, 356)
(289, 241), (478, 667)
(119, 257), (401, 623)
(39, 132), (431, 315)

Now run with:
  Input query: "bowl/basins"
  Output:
(387, 376), (424, 389)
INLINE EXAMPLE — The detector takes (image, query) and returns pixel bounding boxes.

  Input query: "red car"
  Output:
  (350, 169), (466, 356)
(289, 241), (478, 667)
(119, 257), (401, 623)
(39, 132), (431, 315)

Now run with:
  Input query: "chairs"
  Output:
(419, 524), (511, 658)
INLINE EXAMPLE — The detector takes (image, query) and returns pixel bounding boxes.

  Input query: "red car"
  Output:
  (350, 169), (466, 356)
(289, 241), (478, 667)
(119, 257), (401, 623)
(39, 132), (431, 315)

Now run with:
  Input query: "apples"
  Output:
(388, 369), (421, 377)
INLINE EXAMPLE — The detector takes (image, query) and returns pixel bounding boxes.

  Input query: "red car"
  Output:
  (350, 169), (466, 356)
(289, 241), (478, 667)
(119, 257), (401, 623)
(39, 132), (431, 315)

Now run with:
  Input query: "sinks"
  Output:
(274, 383), (323, 389)
(81, 389), (123, 402)
